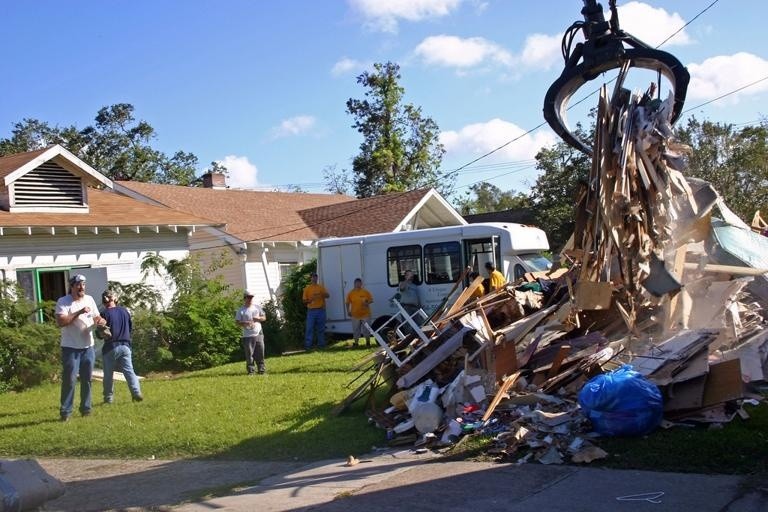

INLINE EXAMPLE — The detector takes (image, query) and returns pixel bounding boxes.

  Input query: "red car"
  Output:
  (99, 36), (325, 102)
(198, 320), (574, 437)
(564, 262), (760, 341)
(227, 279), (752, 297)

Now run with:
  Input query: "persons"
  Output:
(235, 290), (266, 374)
(484, 261), (506, 289)
(303, 273), (330, 349)
(96, 290), (143, 403)
(54, 274), (106, 422)
(345, 278), (374, 349)
(469, 271), (485, 300)
(397, 270), (423, 334)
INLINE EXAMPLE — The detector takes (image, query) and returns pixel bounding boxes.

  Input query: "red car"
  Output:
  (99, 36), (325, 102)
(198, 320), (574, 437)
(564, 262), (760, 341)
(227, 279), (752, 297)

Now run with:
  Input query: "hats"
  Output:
(242, 289), (254, 298)
(68, 274), (86, 295)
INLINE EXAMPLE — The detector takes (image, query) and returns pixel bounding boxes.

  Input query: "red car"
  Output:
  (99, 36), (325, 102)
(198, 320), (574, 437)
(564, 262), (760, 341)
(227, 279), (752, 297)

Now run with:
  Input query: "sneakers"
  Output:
(365, 344), (371, 349)
(132, 395), (143, 402)
(61, 415), (70, 422)
(352, 342), (359, 349)
(81, 412), (90, 418)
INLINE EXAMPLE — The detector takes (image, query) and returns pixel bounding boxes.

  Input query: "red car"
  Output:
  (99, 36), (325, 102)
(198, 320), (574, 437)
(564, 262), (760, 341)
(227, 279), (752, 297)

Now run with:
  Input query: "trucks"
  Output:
(316, 221), (554, 345)
